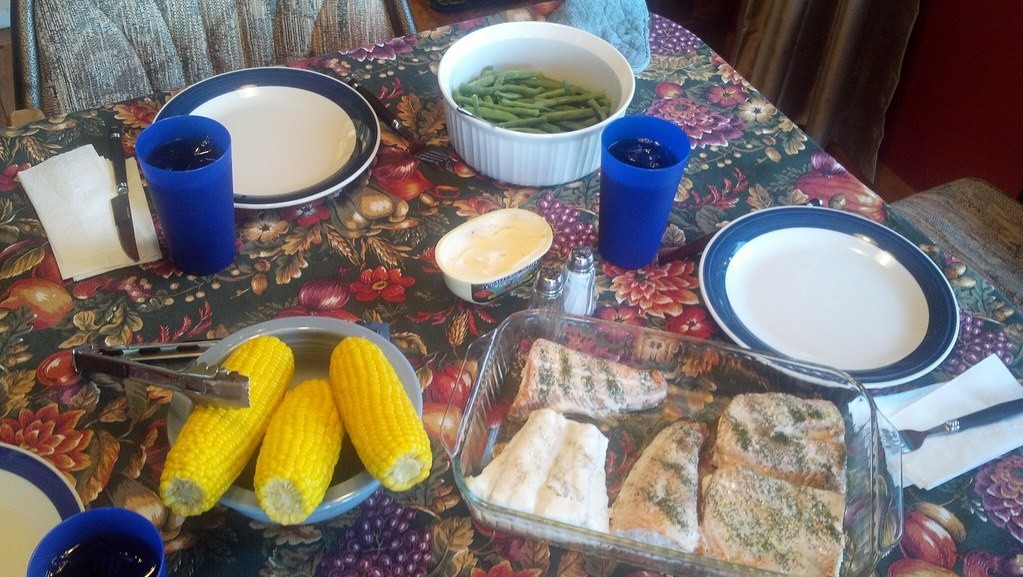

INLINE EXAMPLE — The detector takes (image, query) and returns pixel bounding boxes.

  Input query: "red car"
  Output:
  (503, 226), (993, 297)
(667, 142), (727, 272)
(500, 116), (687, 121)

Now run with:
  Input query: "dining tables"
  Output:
(0, 0), (1023, 577)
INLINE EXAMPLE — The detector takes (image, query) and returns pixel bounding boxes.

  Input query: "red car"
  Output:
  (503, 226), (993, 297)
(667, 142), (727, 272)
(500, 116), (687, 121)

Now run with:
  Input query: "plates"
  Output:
(0, 441), (85, 577)
(151, 66), (382, 210)
(698, 205), (960, 389)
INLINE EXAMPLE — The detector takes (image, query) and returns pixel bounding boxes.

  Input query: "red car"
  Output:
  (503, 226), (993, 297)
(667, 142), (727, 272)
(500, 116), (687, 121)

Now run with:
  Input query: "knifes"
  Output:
(109, 125), (140, 263)
(656, 198), (823, 266)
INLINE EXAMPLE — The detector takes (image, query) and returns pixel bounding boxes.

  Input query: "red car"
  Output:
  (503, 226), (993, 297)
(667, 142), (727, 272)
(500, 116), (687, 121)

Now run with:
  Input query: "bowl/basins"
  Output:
(438, 21), (636, 187)
(168, 316), (423, 526)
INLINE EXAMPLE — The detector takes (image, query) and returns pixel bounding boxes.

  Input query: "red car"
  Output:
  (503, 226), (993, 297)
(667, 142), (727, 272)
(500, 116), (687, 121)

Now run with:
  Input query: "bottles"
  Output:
(528, 246), (597, 340)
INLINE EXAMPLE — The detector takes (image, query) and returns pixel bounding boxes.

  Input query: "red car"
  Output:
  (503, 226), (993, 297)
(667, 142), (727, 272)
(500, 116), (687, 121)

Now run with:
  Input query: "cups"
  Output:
(135, 115), (236, 276)
(26, 507), (169, 577)
(598, 114), (690, 270)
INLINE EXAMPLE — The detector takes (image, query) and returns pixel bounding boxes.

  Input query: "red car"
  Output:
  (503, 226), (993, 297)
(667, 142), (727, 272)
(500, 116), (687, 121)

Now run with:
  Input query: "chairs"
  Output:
(889, 177), (1022, 309)
(8, 0), (416, 128)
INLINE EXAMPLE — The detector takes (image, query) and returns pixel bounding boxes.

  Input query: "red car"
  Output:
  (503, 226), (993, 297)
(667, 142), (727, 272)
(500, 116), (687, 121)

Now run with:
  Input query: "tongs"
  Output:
(71, 338), (251, 411)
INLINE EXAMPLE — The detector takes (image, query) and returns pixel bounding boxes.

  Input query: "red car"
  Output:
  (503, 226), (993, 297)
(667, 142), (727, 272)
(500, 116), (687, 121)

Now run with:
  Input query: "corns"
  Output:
(158, 335), (294, 517)
(330, 336), (433, 493)
(253, 378), (343, 525)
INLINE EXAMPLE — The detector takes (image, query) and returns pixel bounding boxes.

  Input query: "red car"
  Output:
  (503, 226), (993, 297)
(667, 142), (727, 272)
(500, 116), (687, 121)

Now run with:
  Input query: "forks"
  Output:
(881, 398), (1023, 454)
(348, 77), (451, 165)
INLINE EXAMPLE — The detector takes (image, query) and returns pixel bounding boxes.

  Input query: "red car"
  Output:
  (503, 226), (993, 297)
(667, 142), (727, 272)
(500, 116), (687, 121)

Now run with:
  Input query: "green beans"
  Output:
(451, 65), (612, 133)
